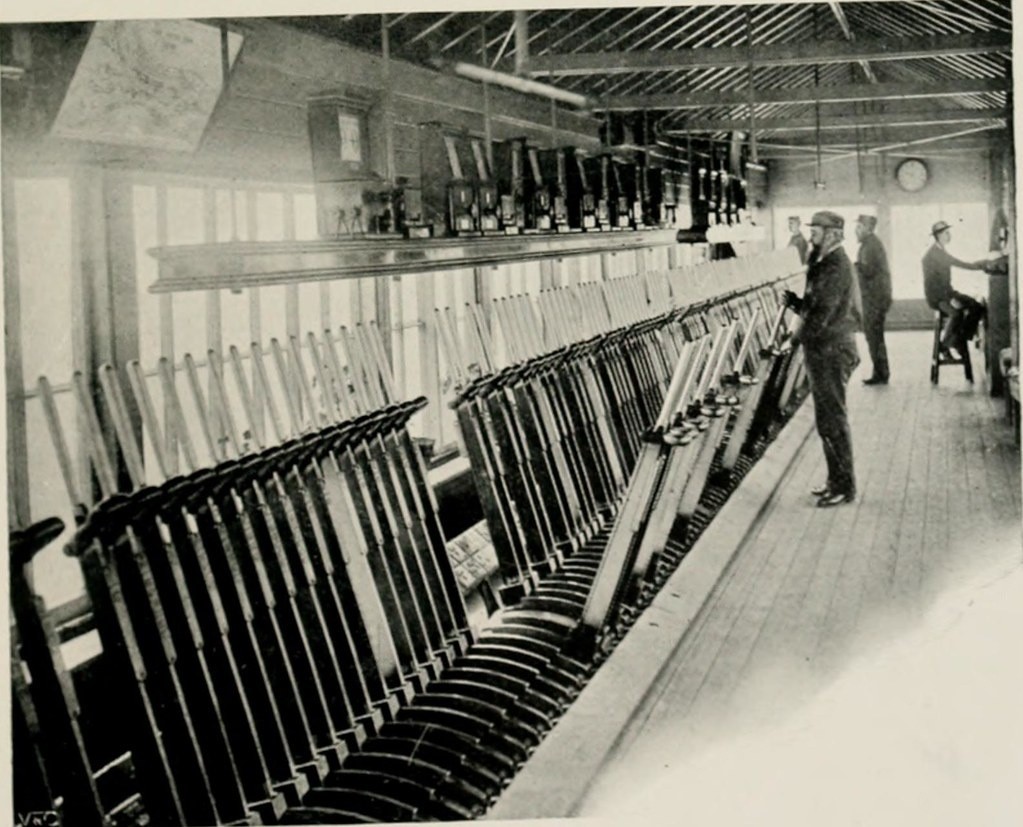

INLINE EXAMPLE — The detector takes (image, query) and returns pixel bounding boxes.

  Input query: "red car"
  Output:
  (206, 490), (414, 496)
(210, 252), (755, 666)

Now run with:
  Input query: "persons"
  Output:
(922, 221), (991, 358)
(780, 212), (858, 505)
(848, 215), (893, 386)
(786, 215), (808, 263)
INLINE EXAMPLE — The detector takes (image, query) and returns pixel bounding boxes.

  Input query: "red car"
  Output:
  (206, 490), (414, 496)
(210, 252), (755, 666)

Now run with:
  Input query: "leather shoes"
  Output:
(817, 490), (855, 506)
(811, 485), (830, 494)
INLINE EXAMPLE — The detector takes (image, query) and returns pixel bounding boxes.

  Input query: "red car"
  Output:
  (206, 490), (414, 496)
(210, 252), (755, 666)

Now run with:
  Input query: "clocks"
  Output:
(893, 157), (931, 192)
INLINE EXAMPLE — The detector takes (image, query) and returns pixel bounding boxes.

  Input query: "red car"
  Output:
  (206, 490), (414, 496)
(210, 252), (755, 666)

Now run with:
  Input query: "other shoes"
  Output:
(939, 341), (954, 361)
(862, 375), (888, 384)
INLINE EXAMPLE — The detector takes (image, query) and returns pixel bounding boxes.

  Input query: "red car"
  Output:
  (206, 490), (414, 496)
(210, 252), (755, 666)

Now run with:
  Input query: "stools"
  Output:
(930, 310), (974, 388)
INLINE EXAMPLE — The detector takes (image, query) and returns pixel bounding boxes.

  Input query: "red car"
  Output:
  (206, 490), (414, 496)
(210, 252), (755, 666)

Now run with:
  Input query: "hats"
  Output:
(805, 211), (843, 228)
(855, 215), (877, 226)
(929, 222), (953, 236)
